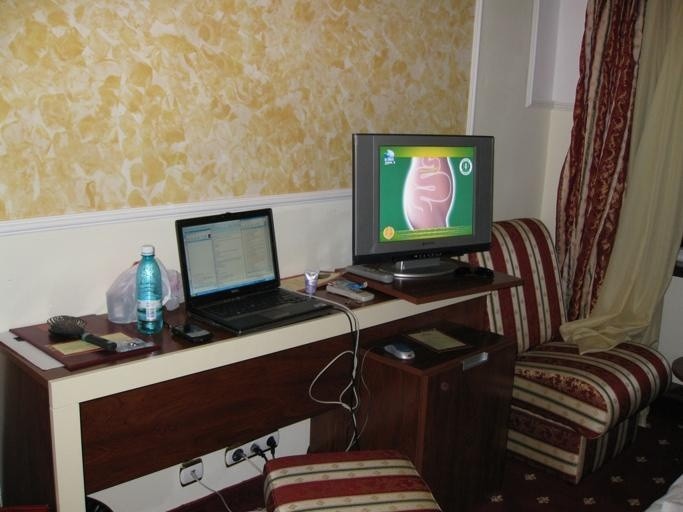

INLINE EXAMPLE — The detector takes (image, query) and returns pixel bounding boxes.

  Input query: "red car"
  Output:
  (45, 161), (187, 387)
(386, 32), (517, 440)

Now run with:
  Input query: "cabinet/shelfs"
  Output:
(351, 319), (518, 512)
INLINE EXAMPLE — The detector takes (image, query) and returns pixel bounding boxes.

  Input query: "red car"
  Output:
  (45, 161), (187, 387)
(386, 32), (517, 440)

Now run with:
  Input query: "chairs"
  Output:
(456, 216), (674, 487)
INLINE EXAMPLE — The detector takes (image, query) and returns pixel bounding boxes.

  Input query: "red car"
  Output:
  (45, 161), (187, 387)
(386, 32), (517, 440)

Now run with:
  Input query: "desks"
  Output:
(0, 258), (526, 512)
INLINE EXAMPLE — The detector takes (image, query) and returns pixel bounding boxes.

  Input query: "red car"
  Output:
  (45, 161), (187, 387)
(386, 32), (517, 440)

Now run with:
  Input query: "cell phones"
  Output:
(173, 324), (213, 346)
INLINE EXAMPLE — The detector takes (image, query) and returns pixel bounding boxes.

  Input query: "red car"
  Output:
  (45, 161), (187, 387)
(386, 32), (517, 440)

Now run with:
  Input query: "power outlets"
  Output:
(180, 460), (204, 486)
(224, 428), (280, 466)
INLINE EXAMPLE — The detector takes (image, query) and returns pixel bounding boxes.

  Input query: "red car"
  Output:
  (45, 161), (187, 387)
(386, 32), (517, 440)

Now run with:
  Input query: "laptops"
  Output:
(174, 208), (333, 337)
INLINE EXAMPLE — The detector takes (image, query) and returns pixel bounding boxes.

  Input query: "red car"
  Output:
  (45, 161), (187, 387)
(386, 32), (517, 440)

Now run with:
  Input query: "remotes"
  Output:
(326, 284), (376, 302)
(344, 265), (395, 284)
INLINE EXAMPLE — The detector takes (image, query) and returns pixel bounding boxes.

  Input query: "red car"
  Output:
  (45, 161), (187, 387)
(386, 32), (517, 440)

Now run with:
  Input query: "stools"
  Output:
(260, 449), (442, 512)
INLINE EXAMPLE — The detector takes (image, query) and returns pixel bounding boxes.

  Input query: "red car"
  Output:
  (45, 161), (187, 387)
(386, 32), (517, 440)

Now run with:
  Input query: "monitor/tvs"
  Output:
(352, 133), (496, 279)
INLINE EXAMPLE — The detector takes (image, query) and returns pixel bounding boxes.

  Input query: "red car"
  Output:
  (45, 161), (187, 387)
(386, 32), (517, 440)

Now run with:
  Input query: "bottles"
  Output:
(135, 245), (162, 334)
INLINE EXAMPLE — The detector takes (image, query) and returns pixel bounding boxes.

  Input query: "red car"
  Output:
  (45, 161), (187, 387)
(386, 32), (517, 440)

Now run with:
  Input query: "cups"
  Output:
(302, 269), (319, 294)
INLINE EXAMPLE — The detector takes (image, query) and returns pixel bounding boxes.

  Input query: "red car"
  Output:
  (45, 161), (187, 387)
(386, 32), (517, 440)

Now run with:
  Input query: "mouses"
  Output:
(384, 344), (416, 360)
